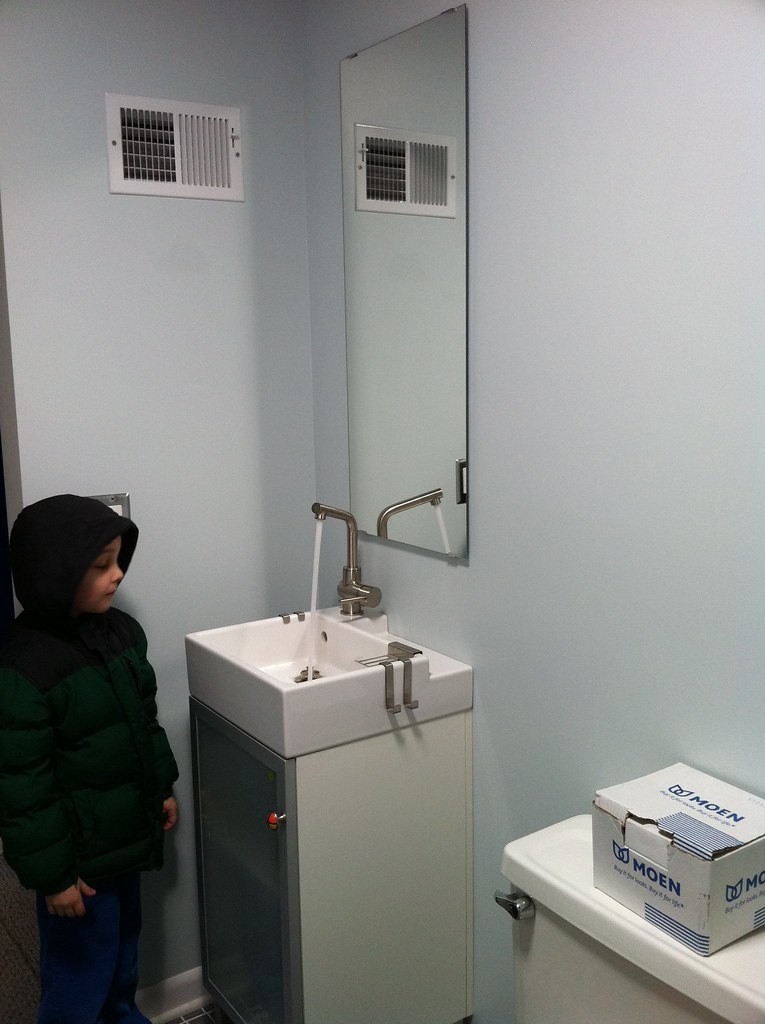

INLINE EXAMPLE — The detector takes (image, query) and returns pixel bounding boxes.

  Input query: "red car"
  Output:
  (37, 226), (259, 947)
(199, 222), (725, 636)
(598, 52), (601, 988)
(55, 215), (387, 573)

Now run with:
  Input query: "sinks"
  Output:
(184, 613), (430, 760)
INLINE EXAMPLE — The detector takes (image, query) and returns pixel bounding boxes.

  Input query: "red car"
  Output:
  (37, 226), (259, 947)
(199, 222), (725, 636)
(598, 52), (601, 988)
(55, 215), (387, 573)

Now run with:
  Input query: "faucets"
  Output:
(311, 502), (382, 617)
(377, 487), (444, 539)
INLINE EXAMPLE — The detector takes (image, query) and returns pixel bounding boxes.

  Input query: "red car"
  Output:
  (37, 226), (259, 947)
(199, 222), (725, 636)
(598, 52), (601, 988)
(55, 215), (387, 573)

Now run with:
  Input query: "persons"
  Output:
(0, 495), (178, 1024)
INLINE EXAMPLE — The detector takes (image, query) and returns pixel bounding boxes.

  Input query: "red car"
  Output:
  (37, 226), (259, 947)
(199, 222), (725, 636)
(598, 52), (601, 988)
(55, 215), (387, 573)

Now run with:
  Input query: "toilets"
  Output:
(494, 811), (765, 1024)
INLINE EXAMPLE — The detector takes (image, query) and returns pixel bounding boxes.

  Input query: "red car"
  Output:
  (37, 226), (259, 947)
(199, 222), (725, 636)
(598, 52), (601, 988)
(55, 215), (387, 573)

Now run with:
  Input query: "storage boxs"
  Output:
(592, 761), (765, 958)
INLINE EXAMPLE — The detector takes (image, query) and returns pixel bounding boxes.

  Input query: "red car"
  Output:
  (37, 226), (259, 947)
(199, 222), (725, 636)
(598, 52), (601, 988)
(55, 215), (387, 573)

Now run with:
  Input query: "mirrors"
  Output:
(338, 3), (470, 561)
